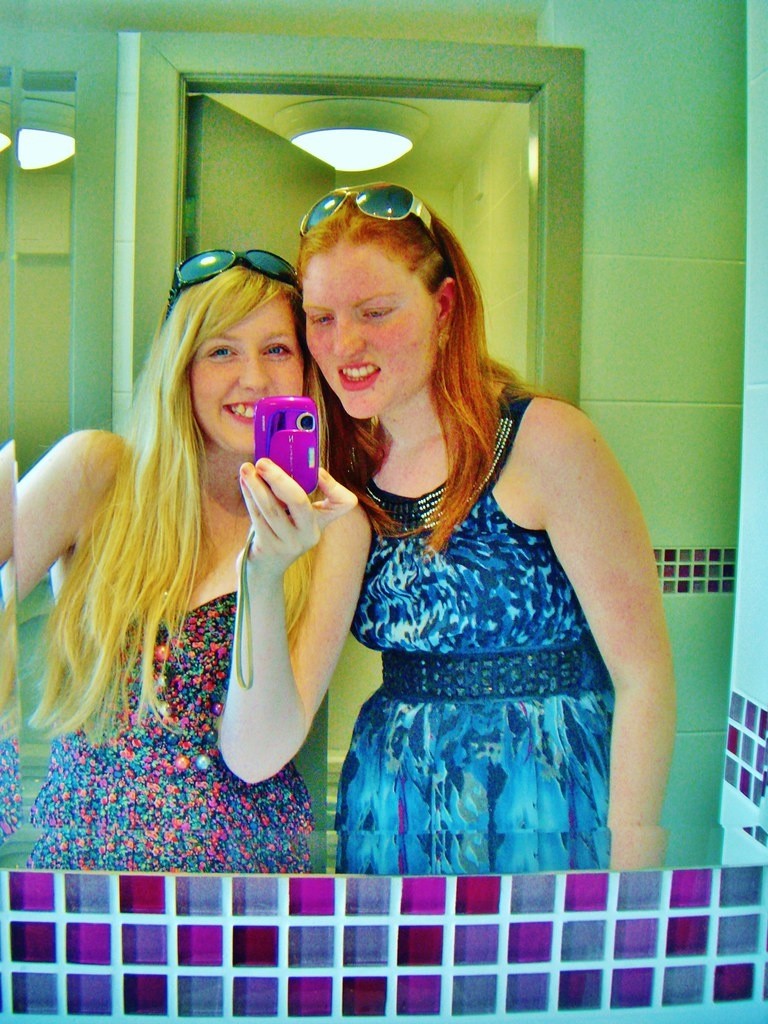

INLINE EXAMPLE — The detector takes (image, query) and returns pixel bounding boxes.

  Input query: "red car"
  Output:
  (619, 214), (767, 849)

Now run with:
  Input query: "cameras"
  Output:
(252, 396), (319, 502)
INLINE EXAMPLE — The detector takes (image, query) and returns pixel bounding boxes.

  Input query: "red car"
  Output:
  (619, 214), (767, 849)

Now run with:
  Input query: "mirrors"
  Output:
(0, 0), (768, 877)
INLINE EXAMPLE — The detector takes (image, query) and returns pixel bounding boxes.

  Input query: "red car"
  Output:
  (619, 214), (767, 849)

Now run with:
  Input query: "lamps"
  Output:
(272, 98), (429, 172)
(17, 99), (77, 171)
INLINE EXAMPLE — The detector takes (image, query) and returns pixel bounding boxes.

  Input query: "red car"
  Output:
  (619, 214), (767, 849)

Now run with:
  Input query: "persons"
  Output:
(297, 184), (675, 873)
(0, 252), (371, 874)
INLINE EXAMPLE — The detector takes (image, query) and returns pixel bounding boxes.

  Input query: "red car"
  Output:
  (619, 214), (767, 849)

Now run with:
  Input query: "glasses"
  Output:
(164, 248), (302, 320)
(299, 180), (447, 265)
